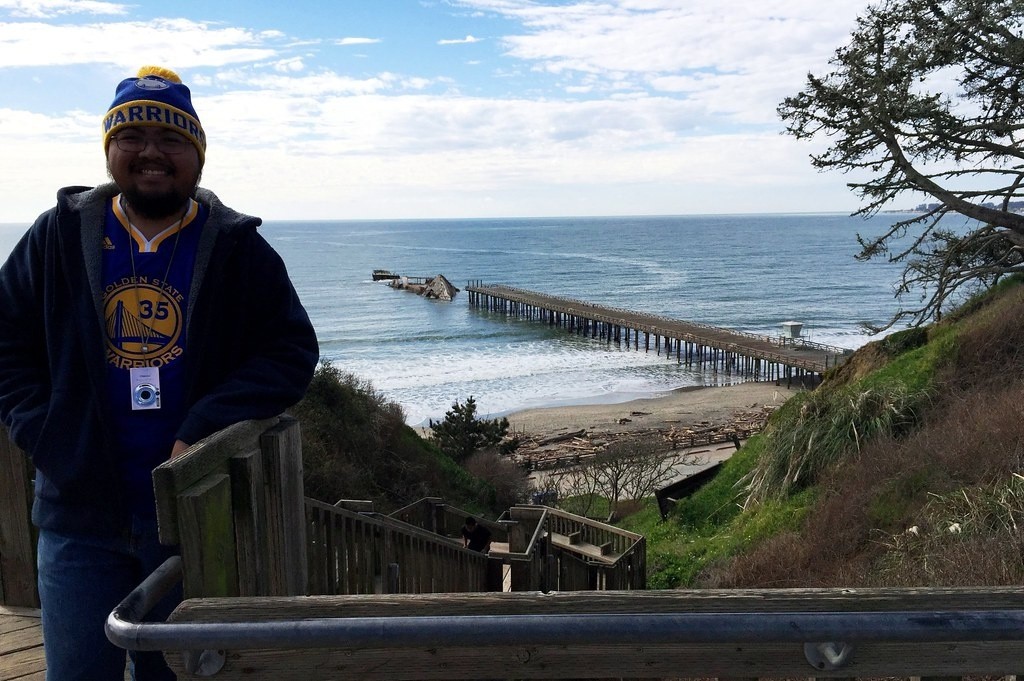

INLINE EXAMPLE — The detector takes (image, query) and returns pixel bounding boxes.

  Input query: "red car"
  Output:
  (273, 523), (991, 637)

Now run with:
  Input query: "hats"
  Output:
(102, 66), (205, 170)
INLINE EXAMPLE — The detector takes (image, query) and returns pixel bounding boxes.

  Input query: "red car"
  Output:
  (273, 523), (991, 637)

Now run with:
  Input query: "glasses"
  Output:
(110, 126), (194, 155)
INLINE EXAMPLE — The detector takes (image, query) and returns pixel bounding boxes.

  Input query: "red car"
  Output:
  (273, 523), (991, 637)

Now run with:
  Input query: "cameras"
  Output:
(130, 367), (161, 410)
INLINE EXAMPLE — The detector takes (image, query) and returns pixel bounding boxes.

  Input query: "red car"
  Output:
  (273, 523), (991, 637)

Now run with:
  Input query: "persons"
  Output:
(462, 517), (493, 554)
(0, 67), (320, 681)
